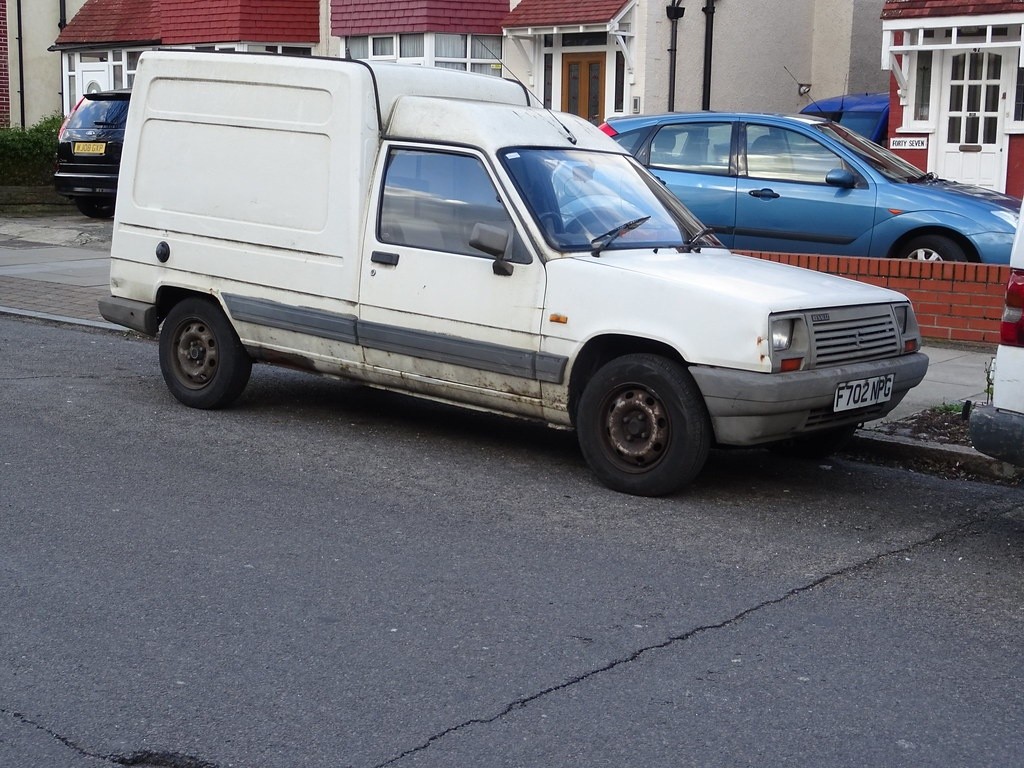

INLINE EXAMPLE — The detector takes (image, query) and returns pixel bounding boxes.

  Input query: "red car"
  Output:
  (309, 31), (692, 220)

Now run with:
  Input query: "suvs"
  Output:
(52, 86), (135, 218)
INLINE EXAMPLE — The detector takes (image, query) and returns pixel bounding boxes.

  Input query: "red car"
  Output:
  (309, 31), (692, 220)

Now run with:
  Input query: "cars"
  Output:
(792, 92), (893, 157)
(549, 111), (1022, 264)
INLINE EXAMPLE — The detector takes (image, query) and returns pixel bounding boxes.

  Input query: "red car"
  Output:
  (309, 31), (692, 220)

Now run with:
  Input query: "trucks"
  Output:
(97, 47), (928, 502)
(966, 194), (1024, 467)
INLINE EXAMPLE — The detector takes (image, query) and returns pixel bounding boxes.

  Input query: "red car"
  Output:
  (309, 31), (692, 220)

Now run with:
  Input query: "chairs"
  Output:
(750, 135), (773, 154)
